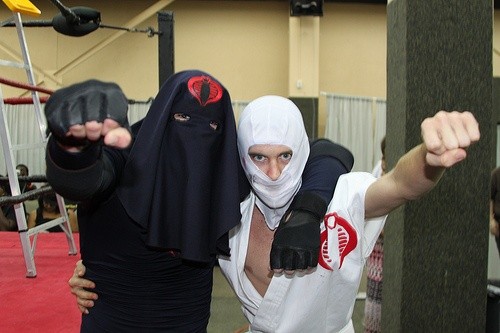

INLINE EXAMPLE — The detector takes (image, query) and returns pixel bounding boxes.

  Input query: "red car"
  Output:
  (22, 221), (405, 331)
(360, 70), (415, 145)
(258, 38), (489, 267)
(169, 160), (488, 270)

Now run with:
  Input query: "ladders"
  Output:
(0, 0), (77, 278)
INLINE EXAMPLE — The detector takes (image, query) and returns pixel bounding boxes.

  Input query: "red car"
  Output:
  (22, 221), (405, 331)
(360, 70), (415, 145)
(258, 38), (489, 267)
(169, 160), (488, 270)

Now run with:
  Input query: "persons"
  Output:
(44, 70), (354, 333)
(69, 96), (480, 333)
(0, 164), (68, 232)
(490, 162), (500, 262)
(365, 136), (385, 333)
